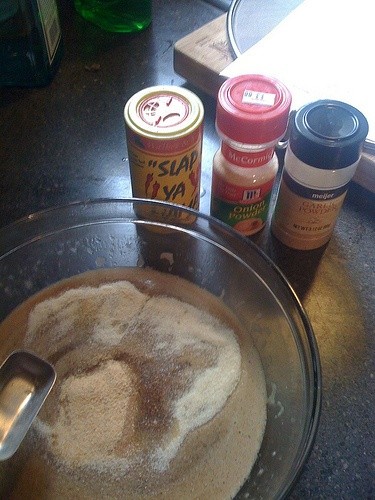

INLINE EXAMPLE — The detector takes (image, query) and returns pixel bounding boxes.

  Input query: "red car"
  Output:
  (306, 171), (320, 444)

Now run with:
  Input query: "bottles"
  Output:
(271, 100), (368, 251)
(74, 0), (152, 33)
(124, 84), (204, 236)
(211, 73), (291, 237)
(0, 0), (64, 87)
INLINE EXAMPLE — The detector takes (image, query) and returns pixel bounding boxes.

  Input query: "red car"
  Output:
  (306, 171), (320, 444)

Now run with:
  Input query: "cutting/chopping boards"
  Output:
(172, 12), (375, 199)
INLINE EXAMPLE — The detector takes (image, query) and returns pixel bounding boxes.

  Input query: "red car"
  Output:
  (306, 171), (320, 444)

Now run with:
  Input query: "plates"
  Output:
(226, 0), (375, 149)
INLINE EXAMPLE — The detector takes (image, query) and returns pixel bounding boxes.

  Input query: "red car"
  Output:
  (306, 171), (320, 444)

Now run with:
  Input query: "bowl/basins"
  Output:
(0, 197), (324, 500)
